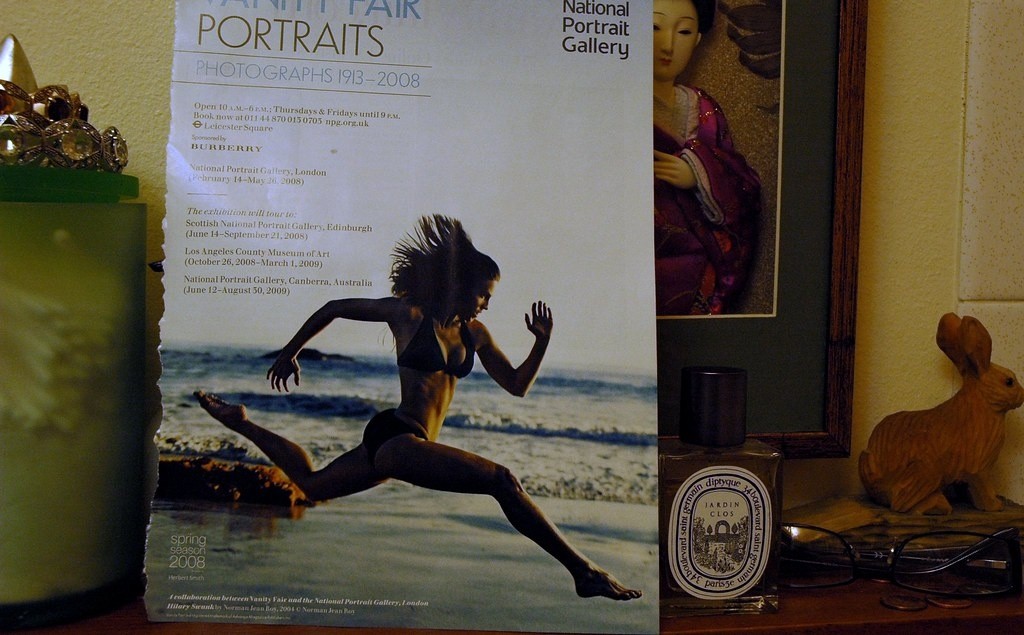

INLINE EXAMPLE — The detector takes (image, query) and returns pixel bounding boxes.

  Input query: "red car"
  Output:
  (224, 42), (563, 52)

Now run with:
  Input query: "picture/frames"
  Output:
(653, 0), (870, 460)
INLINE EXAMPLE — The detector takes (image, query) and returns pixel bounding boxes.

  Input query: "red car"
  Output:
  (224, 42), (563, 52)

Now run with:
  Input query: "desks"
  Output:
(661, 573), (1024, 635)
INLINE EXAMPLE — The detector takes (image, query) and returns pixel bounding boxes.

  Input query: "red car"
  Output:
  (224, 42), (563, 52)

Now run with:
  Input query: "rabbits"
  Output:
(857, 313), (1024, 516)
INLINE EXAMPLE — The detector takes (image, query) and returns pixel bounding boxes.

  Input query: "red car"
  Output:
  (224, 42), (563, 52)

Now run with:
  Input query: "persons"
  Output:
(653, 1), (760, 316)
(194, 215), (643, 600)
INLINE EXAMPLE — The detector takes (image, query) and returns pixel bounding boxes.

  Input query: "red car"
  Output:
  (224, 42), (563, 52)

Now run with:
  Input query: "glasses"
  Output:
(769, 522), (1022, 598)
